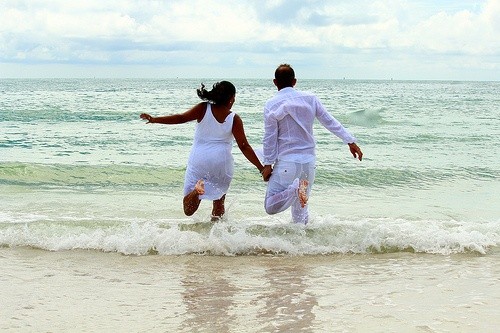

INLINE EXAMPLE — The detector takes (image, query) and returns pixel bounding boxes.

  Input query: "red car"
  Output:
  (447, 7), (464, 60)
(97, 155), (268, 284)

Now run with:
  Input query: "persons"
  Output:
(139, 80), (271, 221)
(260, 63), (363, 226)
